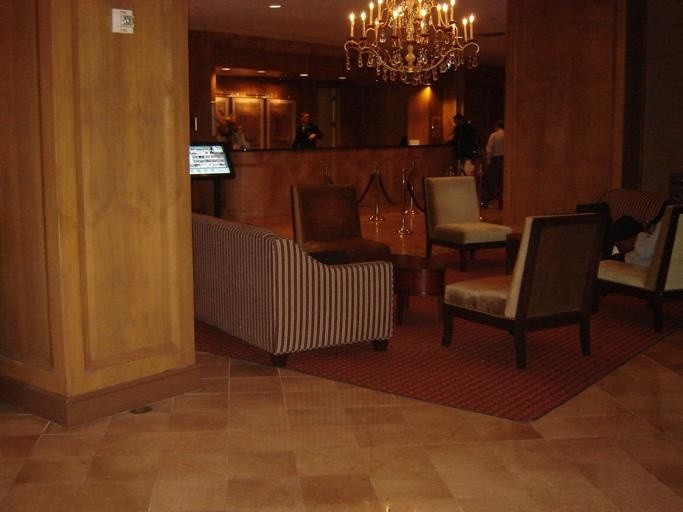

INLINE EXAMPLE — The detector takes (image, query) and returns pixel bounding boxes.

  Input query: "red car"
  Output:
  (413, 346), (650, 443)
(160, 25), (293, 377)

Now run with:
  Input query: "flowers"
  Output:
(213, 110), (245, 140)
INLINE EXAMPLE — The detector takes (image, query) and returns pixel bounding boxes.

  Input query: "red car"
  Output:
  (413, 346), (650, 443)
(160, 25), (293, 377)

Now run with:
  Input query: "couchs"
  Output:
(193, 215), (396, 366)
(596, 189), (669, 231)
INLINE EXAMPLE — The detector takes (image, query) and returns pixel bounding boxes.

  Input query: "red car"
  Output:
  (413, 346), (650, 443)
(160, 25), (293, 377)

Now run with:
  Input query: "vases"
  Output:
(222, 136), (235, 154)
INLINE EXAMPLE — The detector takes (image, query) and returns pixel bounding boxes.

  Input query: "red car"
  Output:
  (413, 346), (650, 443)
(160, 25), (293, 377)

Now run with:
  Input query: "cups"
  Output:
(401, 208), (416, 233)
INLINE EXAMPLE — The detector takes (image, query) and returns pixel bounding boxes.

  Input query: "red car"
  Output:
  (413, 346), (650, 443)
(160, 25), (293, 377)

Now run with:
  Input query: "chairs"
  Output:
(423, 176), (515, 275)
(440, 212), (603, 369)
(592, 205), (683, 330)
(290, 183), (393, 260)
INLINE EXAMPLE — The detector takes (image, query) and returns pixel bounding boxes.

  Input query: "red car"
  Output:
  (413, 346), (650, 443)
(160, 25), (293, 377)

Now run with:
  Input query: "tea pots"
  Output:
(389, 227), (418, 244)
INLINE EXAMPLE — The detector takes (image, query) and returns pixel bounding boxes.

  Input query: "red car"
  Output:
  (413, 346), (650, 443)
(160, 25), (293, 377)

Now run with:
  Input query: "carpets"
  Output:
(195, 248), (682, 422)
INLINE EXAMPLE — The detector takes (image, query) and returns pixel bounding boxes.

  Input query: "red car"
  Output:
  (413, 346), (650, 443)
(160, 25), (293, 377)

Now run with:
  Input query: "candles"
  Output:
(444, 12), (449, 26)
(453, 24), (456, 35)
(394, 16), (399, 30)
(377, 3), (382, 19)
(438, 10), (442, 26)
(470, 23), (474, 39)
(450, 5), (455, 21)
(420, 14), (427, 32)
(350, 20), (355, 37)
(369, 8), (374, 23)
(463, 24), (468, 43)
(362, 20), (367, 38)
(375, 26), (379, 40)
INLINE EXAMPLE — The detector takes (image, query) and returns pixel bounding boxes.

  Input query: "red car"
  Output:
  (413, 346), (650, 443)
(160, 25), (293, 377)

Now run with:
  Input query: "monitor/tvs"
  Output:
(189, 139), (234, 181)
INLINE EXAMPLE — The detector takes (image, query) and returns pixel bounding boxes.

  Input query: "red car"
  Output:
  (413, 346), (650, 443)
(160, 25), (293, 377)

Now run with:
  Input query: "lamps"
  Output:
(343, 0), (480, 87)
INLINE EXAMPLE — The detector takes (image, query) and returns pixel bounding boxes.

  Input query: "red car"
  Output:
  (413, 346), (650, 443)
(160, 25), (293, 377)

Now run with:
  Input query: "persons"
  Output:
(447, 113), (475, 169)
(291, 111), (322, 149)
(479, 119), (504, 209)
